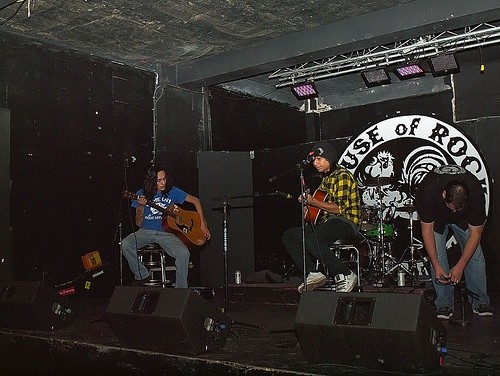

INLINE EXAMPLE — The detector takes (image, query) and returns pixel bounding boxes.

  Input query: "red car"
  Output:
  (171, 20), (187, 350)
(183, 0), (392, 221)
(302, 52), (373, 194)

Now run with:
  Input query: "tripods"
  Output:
(369, 181), (427, 284)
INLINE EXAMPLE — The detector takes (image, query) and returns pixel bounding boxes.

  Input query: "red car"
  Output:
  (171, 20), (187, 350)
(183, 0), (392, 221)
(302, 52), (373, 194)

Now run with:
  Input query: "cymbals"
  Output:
(396, 207), (417, 213)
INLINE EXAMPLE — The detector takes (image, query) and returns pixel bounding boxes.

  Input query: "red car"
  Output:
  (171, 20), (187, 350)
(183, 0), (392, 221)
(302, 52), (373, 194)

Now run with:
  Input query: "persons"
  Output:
(282, 141), (362, 292)
(415, 164), (494, 319)
(122, 164), (211, 289)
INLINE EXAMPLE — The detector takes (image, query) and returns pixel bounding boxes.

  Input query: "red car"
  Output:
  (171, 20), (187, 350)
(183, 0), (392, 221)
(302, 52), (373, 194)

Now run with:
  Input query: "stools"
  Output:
(316, 236), (360, 292)
(137, 243), (177, 289)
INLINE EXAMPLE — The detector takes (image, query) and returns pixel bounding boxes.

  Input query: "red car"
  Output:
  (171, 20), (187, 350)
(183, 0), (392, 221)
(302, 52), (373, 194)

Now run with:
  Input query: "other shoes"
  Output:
(133, 275), (151, 287)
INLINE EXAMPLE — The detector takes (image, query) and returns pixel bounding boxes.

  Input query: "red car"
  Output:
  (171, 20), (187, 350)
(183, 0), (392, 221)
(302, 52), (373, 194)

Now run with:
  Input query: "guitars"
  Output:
(302, 188), (329, 222)
(124, 192), (206, 246)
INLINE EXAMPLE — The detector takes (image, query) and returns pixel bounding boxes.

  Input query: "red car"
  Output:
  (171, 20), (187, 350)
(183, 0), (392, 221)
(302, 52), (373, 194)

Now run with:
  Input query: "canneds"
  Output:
(398, 270), (405, 286)
(235, 270), (241, 284)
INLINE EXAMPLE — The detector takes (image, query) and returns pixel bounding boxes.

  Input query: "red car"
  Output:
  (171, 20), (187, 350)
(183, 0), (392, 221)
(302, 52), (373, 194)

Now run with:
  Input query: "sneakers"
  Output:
(472, 304), (492, 316)
(334, 271), (357, 292)
(437, 307), (454, 319)
(298, 270), (326, 293)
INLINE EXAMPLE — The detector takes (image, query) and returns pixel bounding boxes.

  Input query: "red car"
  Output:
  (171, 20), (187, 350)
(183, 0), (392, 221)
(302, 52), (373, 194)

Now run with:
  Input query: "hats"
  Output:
(312, 142), (337, 162)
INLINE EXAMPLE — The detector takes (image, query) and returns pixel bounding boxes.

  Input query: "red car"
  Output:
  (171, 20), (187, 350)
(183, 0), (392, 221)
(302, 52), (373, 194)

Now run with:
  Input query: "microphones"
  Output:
(309, 147), (324, 156)
(276, 191), (291, 199)
(125, 156), (136, 163)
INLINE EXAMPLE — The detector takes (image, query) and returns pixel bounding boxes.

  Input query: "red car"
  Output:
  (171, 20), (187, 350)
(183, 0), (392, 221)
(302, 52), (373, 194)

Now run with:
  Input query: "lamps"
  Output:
(476, 38), (485, 75)
(290, 81), (319, 101)
(360, 63), (392, 89)
(427, 52), (461, 78)
(392, 59), (426, 81)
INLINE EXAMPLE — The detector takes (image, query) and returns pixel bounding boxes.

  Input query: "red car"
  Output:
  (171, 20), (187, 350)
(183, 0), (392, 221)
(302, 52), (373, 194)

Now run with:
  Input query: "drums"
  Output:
(364, 204), (397, 241)
(361, 205), (376, 231)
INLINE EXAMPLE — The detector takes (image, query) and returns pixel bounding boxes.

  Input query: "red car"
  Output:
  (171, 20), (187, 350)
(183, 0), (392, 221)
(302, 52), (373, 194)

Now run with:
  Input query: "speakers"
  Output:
(0, 281), (78, 330)
(107, 284), (232, 356)
(194, 150), (257, 283)
(295, 290), (447, 374)
